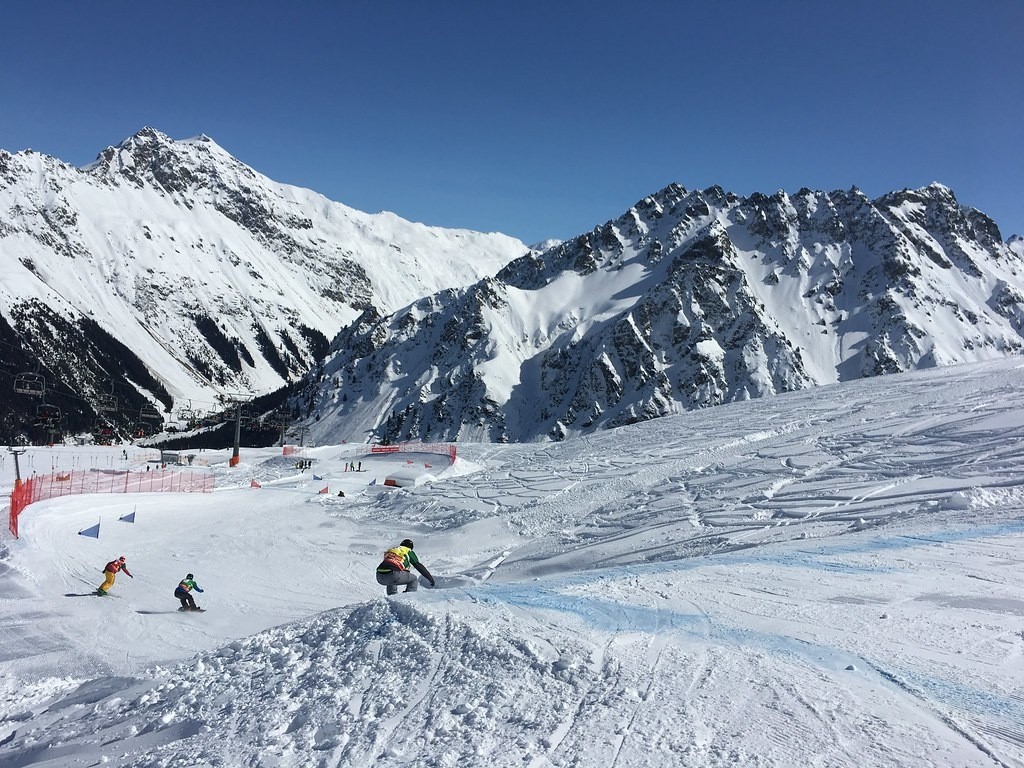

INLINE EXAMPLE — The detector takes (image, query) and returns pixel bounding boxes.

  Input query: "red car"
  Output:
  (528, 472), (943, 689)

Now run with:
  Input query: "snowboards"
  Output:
(178, 605), (207, 613)
(92, 588), (108, 597)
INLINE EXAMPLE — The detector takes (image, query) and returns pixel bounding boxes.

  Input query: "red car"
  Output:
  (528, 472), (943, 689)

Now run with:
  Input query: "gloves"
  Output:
(430, 582), (435, 586)
(102, 570), (105, 574)
(202, 589), (203, 592)
(130, 575), (133, 578)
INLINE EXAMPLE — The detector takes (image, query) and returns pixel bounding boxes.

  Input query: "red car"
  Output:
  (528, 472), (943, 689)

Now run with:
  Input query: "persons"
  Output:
(344, 460), (362, 472)
(376, 539), (435, 596)
(123, 449), (127, 460)
(174, 574), (204, 612)
(147, 463), (167, 471)
(97, 555), (133, 596)
(294, 458), (312, 469)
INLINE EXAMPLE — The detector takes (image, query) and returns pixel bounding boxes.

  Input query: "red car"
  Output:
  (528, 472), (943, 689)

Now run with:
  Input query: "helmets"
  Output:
(119, 556), (126, 561)
(186, 574), (193, 580)
(400, 539), (414, 550)
